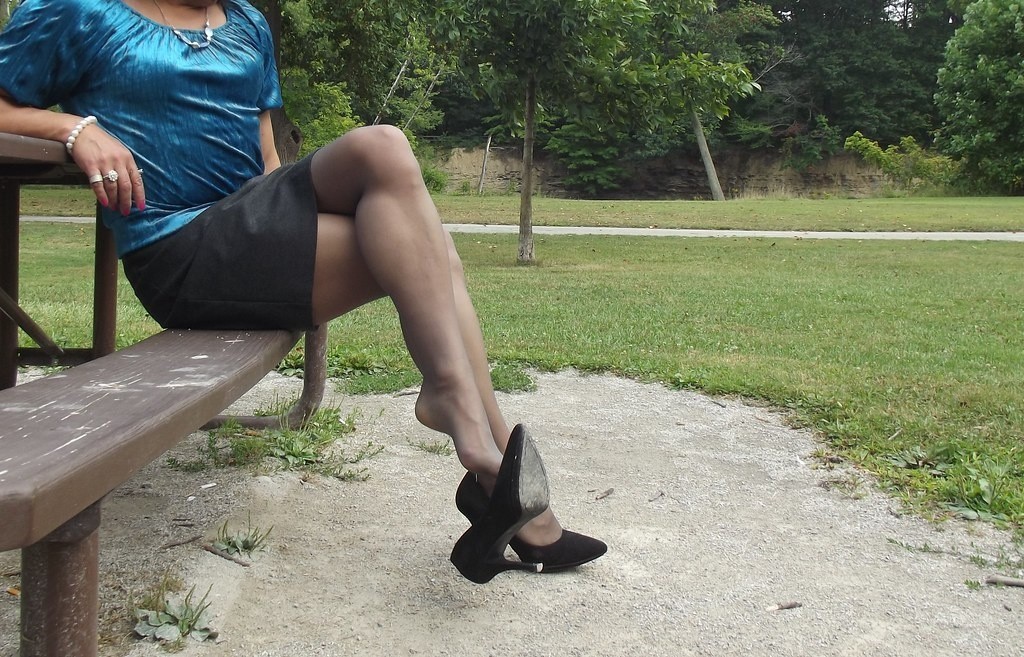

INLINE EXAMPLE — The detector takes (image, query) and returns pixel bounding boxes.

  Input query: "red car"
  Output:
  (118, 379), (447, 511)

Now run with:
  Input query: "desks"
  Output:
(0, 130), (120, 391)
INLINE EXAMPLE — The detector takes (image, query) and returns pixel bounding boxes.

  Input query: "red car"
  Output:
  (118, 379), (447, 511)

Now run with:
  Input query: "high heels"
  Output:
(455, 471), (608, 572)
(450, 423), (551, 584)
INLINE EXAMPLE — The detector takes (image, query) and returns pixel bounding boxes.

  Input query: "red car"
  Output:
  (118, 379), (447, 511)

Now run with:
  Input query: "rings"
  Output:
(89, 175), (103, 184)
(103, 169), (119, 182)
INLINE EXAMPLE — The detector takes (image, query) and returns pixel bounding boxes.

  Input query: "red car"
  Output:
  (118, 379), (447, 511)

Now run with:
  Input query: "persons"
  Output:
(0, 0), (608, 584)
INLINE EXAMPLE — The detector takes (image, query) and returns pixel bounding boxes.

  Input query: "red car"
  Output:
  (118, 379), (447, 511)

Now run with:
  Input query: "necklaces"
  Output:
(154, 0), (213, 49)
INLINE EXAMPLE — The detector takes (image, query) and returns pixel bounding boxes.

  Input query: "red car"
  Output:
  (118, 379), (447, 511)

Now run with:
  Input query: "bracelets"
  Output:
(66, 115), (97, 153)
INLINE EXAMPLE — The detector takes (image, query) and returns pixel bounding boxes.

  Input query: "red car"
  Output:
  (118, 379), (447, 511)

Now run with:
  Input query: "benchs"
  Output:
(0, 313), (329, 657)
